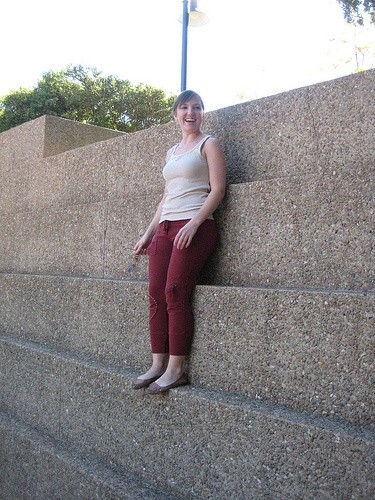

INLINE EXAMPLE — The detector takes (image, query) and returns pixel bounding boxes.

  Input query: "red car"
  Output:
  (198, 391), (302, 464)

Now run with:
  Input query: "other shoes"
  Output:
(147, 370), (187, 394)
(131, 371), (162, 389)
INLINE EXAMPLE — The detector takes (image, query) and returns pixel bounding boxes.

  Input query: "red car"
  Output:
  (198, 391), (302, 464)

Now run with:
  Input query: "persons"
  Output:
(130, 92), (226, 394)
